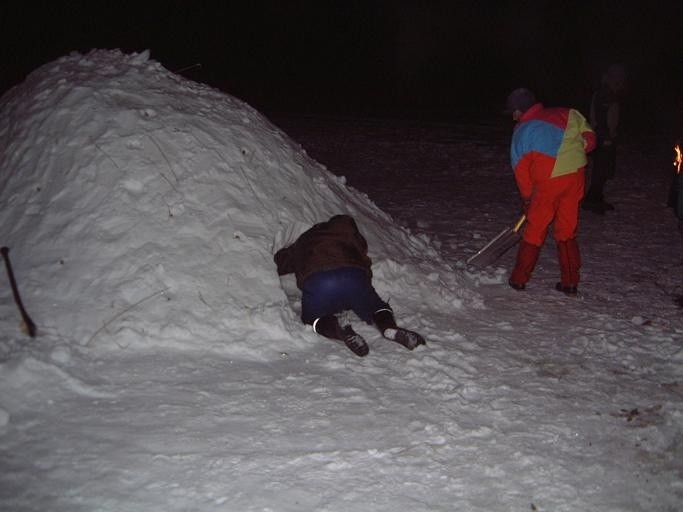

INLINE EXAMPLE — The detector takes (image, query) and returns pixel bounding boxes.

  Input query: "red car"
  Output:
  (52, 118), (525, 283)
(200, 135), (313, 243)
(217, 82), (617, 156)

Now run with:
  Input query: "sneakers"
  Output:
(581, 200), (616, 216)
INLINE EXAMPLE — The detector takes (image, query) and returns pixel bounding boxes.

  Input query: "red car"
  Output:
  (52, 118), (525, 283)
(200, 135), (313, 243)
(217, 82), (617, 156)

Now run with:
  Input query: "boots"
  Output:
(372, 307), (426, 351)
(507, 238), (542, 291)
(554, 236), (582, 298)
(311, 314), (370, 358)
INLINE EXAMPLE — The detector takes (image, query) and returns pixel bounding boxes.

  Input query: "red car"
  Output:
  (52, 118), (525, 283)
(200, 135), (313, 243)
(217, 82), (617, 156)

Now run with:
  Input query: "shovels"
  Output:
(467, 212), (527, 269)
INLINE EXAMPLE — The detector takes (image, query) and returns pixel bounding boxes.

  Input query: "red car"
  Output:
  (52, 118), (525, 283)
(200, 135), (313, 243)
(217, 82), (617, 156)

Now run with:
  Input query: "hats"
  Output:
(506, 87), (537, 115)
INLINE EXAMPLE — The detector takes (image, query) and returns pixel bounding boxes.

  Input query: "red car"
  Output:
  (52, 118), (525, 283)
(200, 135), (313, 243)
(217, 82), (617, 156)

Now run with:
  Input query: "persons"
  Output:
(581, 62), (627, 215)
(505, 88), (598, 297)
(274, 214), (425, 356)
